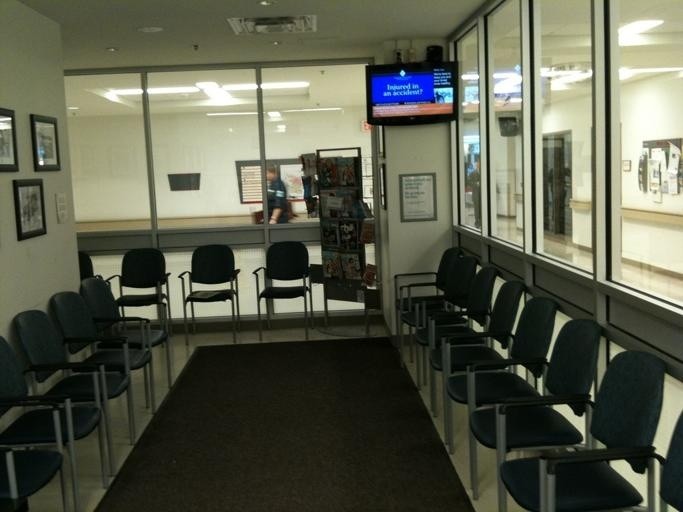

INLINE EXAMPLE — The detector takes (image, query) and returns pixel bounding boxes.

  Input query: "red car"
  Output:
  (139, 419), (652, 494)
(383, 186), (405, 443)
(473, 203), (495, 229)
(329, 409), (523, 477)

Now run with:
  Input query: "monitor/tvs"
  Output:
(365, 60), (459, 126)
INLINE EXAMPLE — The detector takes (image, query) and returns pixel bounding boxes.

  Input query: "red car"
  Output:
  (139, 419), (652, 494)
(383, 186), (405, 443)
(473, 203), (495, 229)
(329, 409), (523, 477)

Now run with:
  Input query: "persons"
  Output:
(467, 144), (478, 164)
(549, 167), (572, 219)
(467, 155), (480, 228)
(260, 164), (288, 224)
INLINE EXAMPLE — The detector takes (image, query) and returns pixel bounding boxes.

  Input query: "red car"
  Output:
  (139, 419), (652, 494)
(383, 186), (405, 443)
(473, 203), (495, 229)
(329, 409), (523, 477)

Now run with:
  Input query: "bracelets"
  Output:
(269, 215), (278, 222)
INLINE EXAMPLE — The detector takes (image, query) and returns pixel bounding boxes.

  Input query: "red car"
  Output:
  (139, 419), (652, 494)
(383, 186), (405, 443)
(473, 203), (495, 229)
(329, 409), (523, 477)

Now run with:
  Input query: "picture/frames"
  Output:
(399, 173), (437, 222)
(12, 178), (47, 241)
(376, 126), (385, 159)
(0, 108), (19, 172)
(377, 163), (386, 211)
(29, 114), (61, 171)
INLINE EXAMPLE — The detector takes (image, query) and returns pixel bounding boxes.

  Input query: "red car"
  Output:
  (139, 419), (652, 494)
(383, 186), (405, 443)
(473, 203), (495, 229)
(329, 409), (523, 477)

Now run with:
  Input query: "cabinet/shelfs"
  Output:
(315, 148), (372, 340)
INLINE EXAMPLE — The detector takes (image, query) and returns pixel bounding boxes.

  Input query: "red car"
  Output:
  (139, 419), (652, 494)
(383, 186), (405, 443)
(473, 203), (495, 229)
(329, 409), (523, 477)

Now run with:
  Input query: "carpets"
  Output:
(91, 337), (477, 511)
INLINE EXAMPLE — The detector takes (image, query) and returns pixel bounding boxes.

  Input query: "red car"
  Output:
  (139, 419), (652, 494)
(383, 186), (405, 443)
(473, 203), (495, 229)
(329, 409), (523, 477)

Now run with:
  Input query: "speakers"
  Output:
(425, 45), (444, 59)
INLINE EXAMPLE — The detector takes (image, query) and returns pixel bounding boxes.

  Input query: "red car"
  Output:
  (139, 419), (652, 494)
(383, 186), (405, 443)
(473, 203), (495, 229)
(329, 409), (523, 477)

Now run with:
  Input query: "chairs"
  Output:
(178, 245), (240, 347)
(105, 248), (171, 347)
(253, 241), (309, 340)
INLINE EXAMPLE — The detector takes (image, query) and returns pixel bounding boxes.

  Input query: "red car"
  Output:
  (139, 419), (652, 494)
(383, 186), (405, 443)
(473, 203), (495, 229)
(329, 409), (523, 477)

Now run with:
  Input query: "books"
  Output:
(301, 153), (376, 281)
